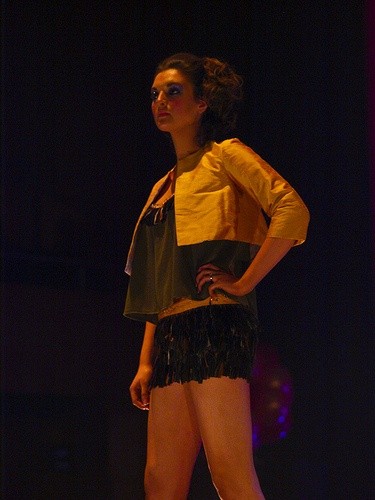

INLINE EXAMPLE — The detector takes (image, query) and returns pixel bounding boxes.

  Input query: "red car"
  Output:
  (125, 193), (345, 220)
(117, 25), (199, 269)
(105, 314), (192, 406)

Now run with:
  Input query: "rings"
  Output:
(209, 272), (213, 280)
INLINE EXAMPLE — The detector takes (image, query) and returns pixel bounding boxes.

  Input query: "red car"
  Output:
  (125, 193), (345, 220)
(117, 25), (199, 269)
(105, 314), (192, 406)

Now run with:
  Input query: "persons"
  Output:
(118, 53), (313, 500)
